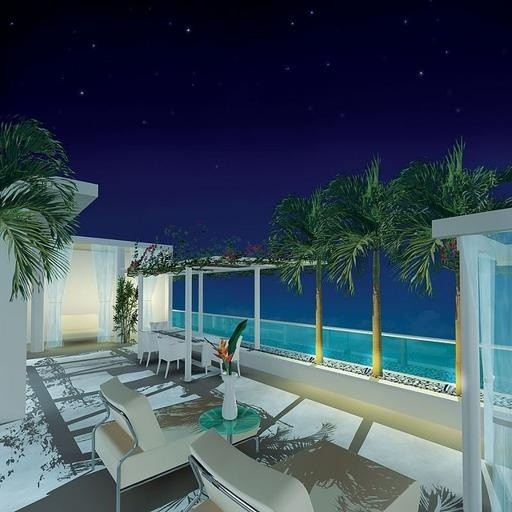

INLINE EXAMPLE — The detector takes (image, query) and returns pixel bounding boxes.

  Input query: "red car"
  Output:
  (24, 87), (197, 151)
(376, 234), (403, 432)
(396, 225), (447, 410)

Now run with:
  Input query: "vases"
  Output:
(222, 372), (239, 420)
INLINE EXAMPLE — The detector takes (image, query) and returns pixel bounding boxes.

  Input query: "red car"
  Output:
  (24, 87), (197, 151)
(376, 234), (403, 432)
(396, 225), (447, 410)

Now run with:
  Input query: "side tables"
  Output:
(199, 406), (260, 453)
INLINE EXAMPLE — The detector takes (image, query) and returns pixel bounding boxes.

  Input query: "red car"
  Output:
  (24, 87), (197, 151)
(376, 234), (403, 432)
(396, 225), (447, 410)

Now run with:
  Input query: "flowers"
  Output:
(203, 319), (248, 375)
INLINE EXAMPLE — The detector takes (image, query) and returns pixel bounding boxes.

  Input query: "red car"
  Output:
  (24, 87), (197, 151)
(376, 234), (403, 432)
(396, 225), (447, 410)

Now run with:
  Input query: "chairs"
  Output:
(182, 430), (418, 510)
(138, 321), (243, 378)
(92, 378), (261, 512)
